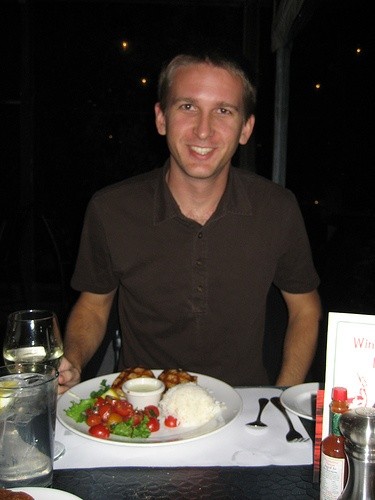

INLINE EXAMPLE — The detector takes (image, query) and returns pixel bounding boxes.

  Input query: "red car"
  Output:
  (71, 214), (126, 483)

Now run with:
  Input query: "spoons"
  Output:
(246, 397), (270, 430)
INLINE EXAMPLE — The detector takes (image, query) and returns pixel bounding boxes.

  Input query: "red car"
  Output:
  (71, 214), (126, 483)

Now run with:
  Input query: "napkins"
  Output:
(52, 386), (314, 470)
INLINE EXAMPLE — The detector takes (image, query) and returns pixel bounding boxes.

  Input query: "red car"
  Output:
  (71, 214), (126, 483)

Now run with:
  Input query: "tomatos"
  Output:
(86, 397), (179, 439)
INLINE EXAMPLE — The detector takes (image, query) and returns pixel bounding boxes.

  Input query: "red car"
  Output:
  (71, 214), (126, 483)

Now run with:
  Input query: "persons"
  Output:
(48, 50), (323, 392)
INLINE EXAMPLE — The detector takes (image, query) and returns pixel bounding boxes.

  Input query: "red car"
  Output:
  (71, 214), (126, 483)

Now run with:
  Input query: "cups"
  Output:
(0, 362), (60, 490)
(122, 377), (166, 412)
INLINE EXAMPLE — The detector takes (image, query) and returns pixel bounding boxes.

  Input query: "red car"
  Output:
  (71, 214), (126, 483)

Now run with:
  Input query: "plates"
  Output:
(280, 382), (319, 421)
(3, 486), (84, 500)
(55, 369), (244, 448)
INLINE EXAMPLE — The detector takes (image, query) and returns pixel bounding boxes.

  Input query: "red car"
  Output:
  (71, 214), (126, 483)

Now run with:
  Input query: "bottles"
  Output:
(319, 386), (349, 499)
(334, 409), (375, 500)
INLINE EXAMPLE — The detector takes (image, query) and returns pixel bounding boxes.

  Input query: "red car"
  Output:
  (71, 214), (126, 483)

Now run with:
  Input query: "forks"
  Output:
(271, 397), (311, 443)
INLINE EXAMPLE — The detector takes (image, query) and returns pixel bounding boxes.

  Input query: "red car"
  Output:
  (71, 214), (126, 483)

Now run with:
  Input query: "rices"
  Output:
(159, 381), (224, 428)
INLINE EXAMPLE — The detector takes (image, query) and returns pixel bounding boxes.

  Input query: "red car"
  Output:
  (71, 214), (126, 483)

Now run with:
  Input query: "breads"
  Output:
(158, 368), (199, 390)
(112, 367), (155, 396)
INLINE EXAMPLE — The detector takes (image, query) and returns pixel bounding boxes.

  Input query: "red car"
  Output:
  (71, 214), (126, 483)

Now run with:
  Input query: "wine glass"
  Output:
(3, 308), (65, 462)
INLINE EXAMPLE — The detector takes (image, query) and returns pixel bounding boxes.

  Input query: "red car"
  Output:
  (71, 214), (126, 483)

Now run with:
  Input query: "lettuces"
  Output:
(64, 380), (152, 439)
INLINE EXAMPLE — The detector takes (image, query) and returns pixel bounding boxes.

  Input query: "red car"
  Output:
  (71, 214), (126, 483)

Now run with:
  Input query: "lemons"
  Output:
(0, 380), (18, 412)
(101, 388), (119, 398)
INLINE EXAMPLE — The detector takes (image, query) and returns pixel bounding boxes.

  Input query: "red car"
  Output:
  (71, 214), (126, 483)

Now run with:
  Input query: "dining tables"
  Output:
(0, 384), (375, 500)
